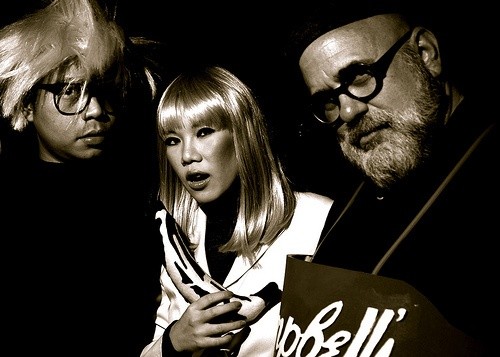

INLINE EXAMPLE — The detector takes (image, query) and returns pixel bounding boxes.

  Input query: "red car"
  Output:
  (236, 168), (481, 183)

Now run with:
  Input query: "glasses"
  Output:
(34, 81), (132, 115)
(308, 28), (414, 126)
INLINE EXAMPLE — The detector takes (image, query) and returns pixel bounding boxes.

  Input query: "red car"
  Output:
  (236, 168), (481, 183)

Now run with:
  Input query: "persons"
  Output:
(282, 0), (500, 357)
(138, 63), (334, 357)
(0, 0), (163, 357)
